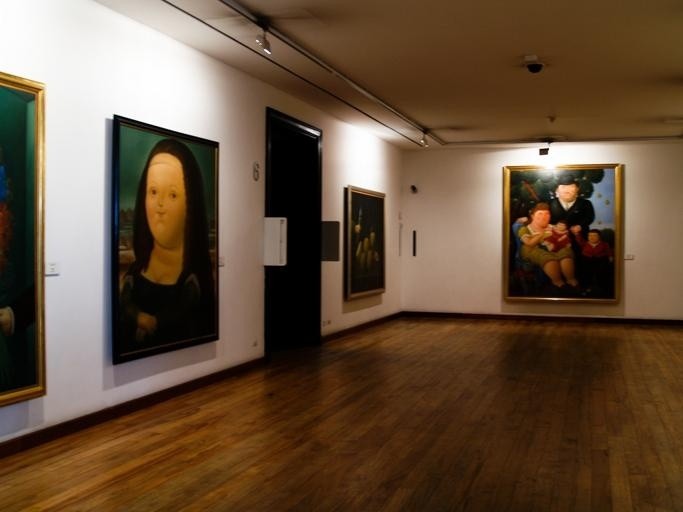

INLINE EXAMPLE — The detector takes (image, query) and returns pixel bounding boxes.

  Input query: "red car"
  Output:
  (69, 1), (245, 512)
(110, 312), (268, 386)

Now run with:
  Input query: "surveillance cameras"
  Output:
(526, 60), (545, 75)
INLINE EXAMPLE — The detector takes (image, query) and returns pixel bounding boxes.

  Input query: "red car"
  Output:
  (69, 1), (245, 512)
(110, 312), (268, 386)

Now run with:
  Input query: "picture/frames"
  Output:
(345, 185), (387, 300)
(1, 72), (46, 408)
(111, 113), (219, 366)
(501, 162), (627, 306)
